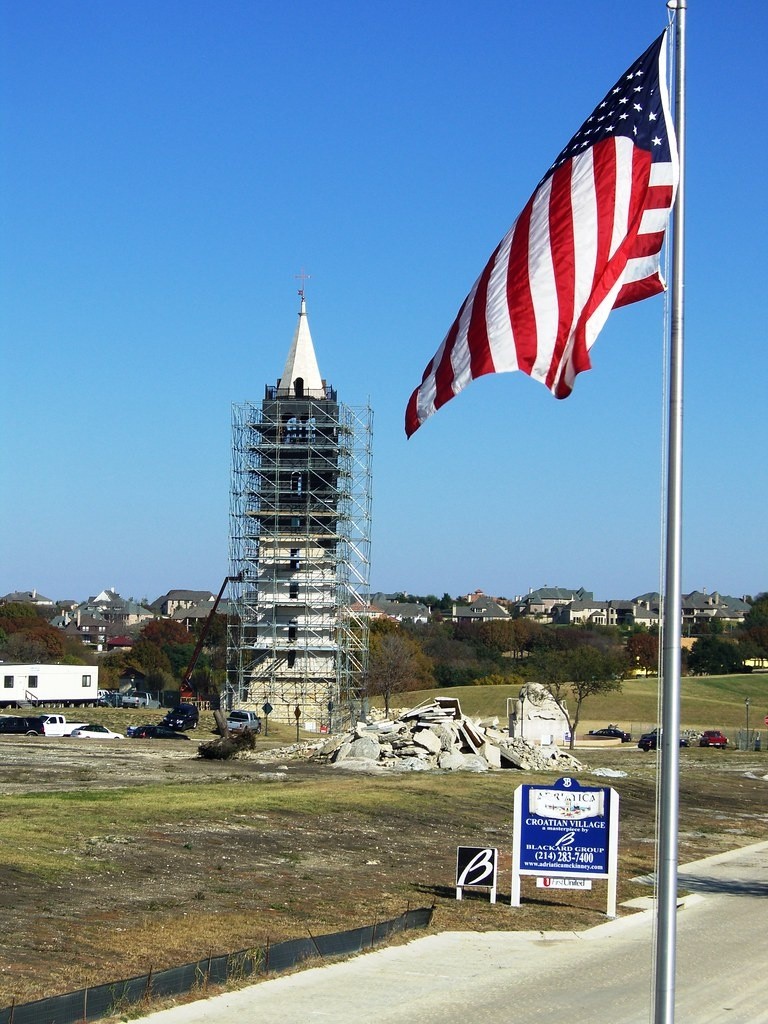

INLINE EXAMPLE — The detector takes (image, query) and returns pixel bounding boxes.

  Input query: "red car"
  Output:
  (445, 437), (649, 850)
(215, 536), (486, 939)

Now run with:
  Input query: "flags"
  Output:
(404, 26), (679, 440)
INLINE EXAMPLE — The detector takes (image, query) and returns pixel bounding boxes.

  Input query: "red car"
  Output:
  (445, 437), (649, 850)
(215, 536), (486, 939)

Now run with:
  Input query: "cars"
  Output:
(132, 725), (190, 740)
(699, 730), (729, 749)
(638, 728), (689, 752)
(585, 729), (631, 743)
(70, 724), (125, 740)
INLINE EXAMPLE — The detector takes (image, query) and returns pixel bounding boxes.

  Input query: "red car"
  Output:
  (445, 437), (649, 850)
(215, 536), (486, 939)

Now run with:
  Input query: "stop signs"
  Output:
(763, 715), (768, 726)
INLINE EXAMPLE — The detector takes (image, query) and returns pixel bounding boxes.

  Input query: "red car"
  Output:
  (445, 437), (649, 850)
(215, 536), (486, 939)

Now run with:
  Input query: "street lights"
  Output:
(744, 697), (751, 747)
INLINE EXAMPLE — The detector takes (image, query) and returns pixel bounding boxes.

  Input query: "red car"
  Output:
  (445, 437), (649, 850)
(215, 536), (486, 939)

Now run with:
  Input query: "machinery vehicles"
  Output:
(177, 569), (250, 708)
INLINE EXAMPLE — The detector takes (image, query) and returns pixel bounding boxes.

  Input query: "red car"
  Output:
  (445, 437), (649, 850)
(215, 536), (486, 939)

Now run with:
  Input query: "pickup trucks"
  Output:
(99, 688), (162, 709)
(224, 709), (261, 735)
(38, 713), (90, 737)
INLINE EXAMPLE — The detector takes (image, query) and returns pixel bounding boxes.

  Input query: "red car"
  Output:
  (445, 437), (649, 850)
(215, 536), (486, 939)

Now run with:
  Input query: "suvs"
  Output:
(163, 703), (199, 732)
(0, 715), (45, 737)
